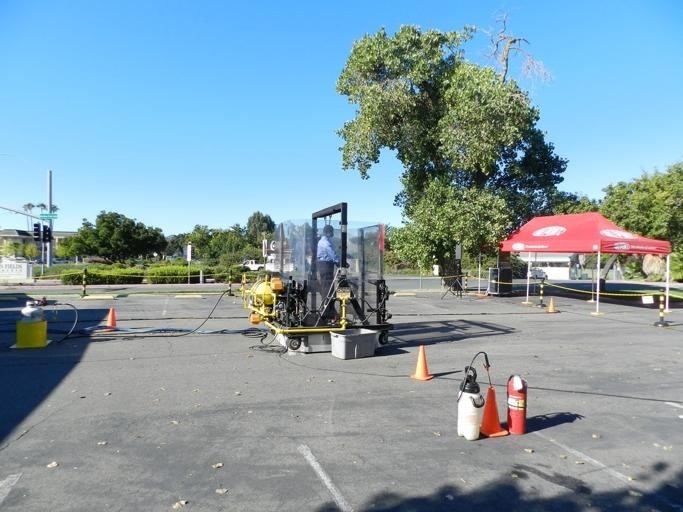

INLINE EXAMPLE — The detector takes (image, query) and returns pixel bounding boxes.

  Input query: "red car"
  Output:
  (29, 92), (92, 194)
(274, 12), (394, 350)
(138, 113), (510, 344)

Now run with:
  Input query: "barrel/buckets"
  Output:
(16, 318), (47, 347)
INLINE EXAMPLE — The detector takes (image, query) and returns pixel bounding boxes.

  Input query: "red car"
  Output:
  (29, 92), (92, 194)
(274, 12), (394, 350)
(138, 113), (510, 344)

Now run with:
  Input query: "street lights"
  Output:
(33, 222), (41, 241)
(43, 226), (51, 243)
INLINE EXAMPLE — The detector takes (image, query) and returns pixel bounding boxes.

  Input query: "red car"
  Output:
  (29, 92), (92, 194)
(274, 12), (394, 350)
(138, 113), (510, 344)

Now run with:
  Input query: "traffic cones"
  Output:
(105, 309), (117, 331)
(545, 298), (559, 314)
(411, 345), (431, 380)
(479, 387), (510, 437)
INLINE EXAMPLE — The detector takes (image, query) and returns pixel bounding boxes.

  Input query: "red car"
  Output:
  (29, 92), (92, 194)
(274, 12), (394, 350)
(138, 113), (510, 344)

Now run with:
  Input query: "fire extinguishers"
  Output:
(506, 374), (527, 435)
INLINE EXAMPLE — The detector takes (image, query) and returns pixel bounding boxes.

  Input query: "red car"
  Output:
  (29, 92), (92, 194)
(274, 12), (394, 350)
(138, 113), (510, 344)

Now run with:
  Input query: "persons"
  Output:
(317, 225), (348, 323)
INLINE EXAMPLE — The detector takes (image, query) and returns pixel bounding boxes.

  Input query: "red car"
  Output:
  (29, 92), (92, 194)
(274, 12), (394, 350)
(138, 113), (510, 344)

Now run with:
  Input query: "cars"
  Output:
(242, 259), (264, 271)
(526, 269), (548, 280)
